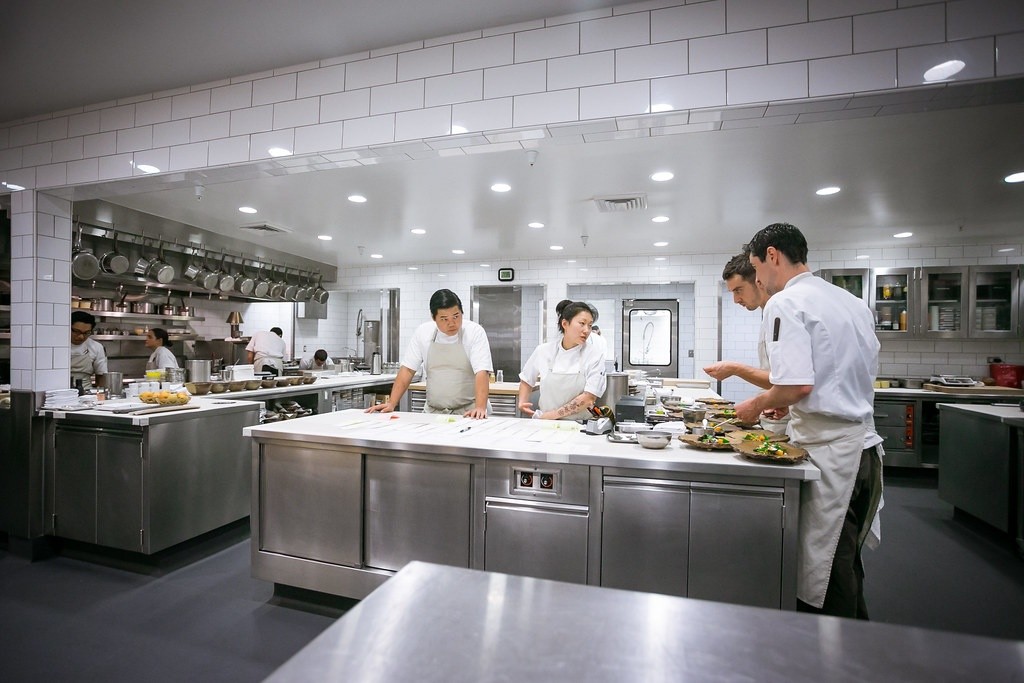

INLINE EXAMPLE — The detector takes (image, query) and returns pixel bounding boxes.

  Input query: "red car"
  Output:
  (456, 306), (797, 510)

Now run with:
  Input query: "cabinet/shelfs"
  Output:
(56, 411), (260, 576)
(0, 305), (206, 340)
(595, 466), (798, 611)
(251, 437), (474, 621)
(813, 264), (1024, 339)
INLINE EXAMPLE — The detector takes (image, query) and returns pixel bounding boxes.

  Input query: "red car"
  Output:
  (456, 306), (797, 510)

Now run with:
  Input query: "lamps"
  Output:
(226, 311), (244, 338)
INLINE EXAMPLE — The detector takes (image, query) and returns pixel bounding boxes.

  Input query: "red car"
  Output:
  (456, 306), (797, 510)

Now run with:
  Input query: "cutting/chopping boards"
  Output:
(923, 383), (1024, 394)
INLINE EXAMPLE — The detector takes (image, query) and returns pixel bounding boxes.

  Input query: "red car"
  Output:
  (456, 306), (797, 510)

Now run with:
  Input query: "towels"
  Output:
(653, 421), (687, 439)
(43, 389), (80, 408)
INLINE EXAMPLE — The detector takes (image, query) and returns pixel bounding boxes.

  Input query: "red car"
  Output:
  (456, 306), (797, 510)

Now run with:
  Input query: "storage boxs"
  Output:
(377, 394), (389, 404)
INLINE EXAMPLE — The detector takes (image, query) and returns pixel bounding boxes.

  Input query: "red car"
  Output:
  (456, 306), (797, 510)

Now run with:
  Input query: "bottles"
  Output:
(75, 378), (92, 396)
(900, 309), (907, 330)
(496, 370), (503, 383)
(489, 373), (495, 383)
(164, 366), (187, 383)
(893, 320), (899, 329)
(372, 352), (381, 375)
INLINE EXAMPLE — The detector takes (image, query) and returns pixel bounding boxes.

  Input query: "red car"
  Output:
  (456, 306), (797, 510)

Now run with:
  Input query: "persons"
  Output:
(71, 310), (108, 388)
(144, 328), (179, 374)
(299, 349), (335, 370)
(364, 289), (495, 420)
(245, 327), (287, 377)
(702, 223), (884, 621)
(518, 300), (607, 422)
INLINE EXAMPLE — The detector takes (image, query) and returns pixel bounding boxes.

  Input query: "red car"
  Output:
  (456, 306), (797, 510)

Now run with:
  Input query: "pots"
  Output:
(72, 224), (329, 316)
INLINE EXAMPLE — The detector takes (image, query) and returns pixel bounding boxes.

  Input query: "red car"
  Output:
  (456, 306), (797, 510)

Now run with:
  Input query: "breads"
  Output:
(139, 390), (189, 405)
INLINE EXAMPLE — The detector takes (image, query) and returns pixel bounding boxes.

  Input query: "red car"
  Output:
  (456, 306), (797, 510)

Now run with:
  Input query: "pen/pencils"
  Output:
(460, 427), (471, 432)
(586, 402), (607, 417)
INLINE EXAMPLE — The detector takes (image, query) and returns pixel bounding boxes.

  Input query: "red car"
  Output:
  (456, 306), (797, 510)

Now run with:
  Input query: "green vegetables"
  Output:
(699, 408), (784, 454)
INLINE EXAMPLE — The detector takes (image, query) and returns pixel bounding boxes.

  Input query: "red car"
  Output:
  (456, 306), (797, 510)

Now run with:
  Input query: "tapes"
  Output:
(597, 418), (605, 425)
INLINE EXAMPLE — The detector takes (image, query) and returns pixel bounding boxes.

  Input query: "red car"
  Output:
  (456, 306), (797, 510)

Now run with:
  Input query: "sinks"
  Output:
(645, 378), (663, 388)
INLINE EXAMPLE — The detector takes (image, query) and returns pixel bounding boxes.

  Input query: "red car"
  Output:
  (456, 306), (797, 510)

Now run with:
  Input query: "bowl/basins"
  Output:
(183, 375), (318, 395)
(660, 395), (682, 405)
(145, 369), (166, 380)
(682, 408), (707, 423)
(635, 430), (675, 449)
(692, 426), (715, 436)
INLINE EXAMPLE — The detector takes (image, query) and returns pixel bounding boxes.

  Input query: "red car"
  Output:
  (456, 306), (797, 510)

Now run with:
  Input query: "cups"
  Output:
(130, 381), (171, 397)
(219, 370), (234, 382)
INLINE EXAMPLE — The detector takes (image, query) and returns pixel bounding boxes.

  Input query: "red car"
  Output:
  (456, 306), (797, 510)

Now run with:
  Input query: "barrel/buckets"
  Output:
(595, 373), (629, 418)
(103, 372), (123, 395)
(185, 359), (212, 383)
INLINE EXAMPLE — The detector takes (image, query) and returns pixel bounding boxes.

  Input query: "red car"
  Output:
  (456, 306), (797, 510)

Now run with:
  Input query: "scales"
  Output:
(929, 374), (975, 387)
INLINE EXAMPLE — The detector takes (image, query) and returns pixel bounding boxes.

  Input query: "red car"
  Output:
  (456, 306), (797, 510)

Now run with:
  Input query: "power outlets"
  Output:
(987, 357), (1001, 362)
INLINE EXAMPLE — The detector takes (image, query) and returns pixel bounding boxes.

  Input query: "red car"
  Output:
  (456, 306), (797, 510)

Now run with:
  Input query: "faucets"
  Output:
(642, 368), (663, 376)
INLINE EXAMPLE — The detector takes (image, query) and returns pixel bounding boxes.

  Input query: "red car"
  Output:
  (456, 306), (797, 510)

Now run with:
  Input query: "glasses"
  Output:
(71, 328), (93, 336)
